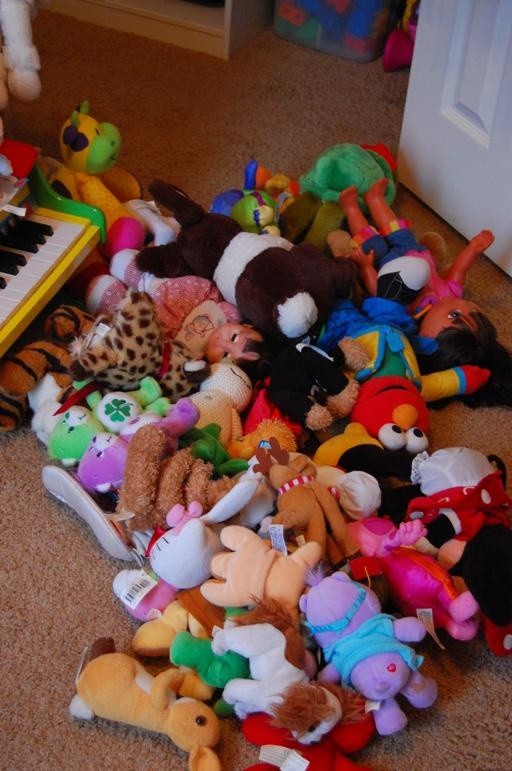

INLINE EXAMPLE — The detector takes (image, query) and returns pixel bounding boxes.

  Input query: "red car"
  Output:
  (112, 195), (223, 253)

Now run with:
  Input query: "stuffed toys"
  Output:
(0, 0), (43, 112)
(1, 100), (512, 770)
(0, 100), (508, 771)
